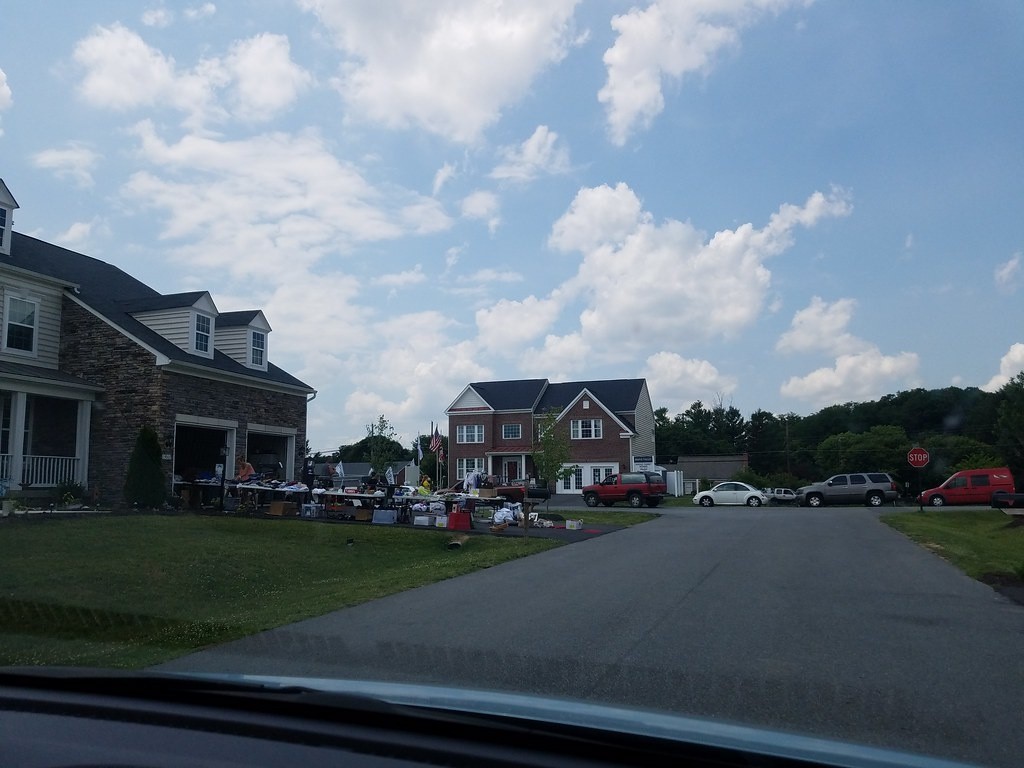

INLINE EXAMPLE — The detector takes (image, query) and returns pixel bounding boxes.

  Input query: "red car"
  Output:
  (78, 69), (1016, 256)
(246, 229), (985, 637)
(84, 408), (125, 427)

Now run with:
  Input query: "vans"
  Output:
(916, 467), (1016, 507)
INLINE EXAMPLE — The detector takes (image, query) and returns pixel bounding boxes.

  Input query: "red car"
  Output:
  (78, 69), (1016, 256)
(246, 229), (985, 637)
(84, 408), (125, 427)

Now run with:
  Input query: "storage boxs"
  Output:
(414, 516), (433, 525)
(434, 515), (447, 527)
(566, 520), (582, 530)
(355, 509), (372, 520)
(272, 501), (297, 515)
(372, 509), (397, 524)
(448, 498), (475, 529)
(301, 504), (325, 516)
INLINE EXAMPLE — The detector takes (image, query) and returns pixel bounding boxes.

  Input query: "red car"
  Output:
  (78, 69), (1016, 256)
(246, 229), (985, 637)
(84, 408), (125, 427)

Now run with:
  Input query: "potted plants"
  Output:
(469, 474), (496, 497)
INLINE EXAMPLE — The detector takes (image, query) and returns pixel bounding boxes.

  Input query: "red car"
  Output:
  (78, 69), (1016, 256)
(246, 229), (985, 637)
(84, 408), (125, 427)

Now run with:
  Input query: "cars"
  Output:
(392, 486), (418, 504)
(795, 472), (897, 507)
(692, 481), (769, 507)
(582, 471), (667, 508)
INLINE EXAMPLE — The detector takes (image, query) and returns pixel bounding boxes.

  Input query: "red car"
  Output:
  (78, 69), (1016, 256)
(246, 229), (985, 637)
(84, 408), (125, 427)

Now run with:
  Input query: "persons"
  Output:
(418, 476), (430, 495)
(235, 456), (256, 508)
(365, 471), (377, 492)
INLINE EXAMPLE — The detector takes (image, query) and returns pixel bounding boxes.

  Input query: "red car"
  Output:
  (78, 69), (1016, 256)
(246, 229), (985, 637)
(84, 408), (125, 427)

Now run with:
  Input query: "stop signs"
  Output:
(908, 448), (929, 467)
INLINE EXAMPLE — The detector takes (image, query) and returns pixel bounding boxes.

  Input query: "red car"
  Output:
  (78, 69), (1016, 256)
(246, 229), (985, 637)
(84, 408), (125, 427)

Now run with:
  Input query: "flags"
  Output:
(417, 436), (424, 460)
(385, 466), (395, 485)
(335, 461), (345, 479)
(429, 429), (442, 451)
(438, 450), (446, 464)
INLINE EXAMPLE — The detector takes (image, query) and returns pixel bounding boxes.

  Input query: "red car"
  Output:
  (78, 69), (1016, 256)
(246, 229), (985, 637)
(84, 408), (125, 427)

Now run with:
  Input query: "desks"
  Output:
(1001, 508), (1024, 528)
(174, 481), (506, 524)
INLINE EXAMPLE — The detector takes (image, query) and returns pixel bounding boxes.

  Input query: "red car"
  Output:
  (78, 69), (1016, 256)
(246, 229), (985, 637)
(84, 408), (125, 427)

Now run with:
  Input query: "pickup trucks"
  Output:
(764, 487), (796, 504)
(434, 475), (525, 507)
